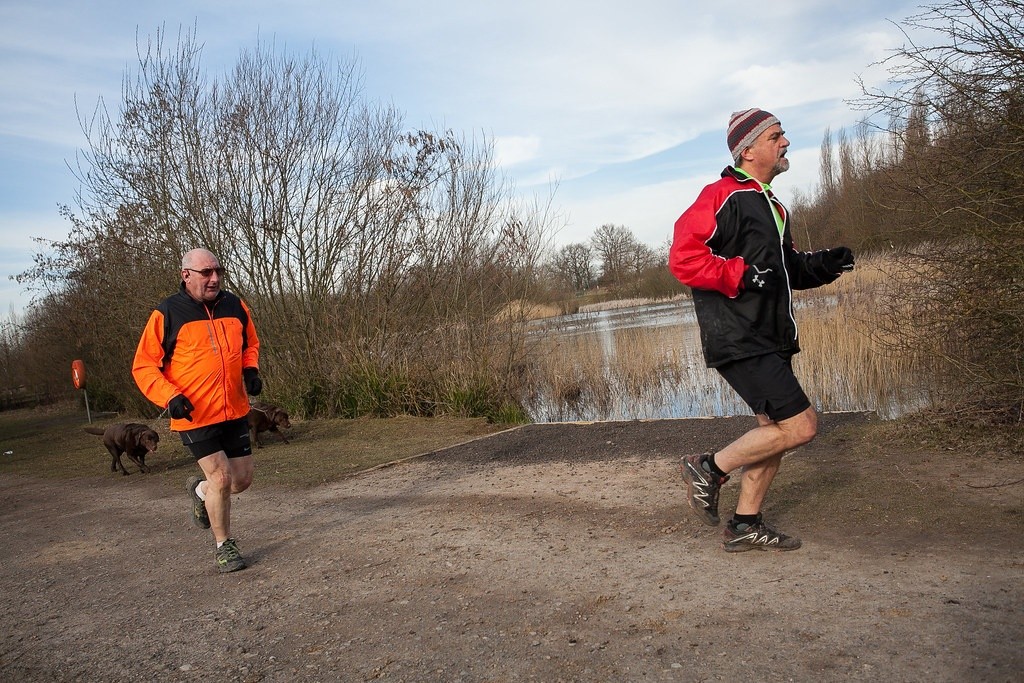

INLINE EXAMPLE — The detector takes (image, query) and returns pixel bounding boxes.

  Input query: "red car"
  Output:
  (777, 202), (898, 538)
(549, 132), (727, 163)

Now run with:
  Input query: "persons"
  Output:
(133, 248), (262, 571)
(668, 109), (855, 552)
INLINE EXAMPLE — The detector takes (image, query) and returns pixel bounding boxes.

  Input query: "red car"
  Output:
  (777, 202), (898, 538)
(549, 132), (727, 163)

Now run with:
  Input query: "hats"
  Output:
(727, 104), (780, 159)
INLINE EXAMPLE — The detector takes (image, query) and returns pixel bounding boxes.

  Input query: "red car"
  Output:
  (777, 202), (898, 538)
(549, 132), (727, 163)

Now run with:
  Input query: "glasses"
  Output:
(182, 267), (227, 277)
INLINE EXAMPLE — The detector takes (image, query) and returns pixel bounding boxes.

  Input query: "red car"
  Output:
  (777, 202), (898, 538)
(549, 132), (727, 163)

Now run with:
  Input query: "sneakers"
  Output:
(721, 519), (803, 552)
(214, 538), (245, 572)
(679, 451), (730, 526)
(186, 476), (213, 529)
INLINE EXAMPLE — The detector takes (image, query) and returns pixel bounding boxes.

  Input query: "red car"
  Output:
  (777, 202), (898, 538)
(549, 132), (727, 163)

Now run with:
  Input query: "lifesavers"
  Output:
(72, 360), (86, 390)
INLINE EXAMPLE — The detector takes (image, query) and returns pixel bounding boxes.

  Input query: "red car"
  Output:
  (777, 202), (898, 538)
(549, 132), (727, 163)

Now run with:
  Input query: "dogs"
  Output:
(85, 422), (160, 475)
(248, 403), (291, 449)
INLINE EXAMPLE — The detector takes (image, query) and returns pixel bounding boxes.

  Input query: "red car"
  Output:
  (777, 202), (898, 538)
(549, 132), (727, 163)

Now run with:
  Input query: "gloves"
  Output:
(243, 366), (262, 396)
(168, 393), (195, 422)
(742, 263), (784, 295)
(820, 245), (857, 279)
